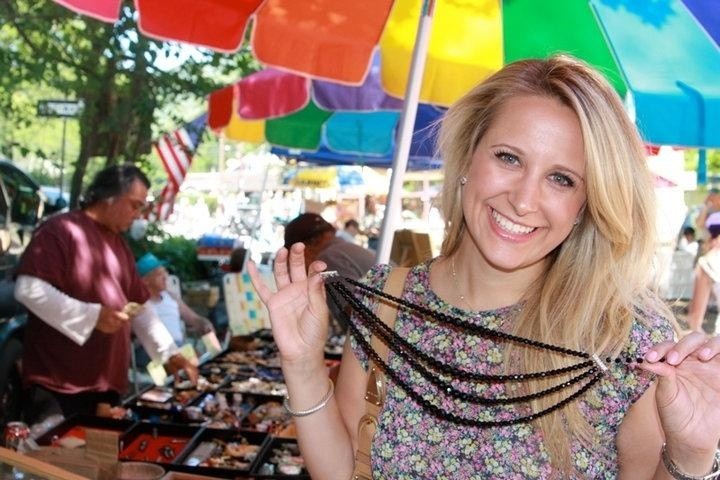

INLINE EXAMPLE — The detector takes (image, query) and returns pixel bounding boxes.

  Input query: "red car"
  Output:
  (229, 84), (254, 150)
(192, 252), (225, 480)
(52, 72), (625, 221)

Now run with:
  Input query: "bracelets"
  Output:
(659, 442), (720, 480)
(282, 378), (335, 417)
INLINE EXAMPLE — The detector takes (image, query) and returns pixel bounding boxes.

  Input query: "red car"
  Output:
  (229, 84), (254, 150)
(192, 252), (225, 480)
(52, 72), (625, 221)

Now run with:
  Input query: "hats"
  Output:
(283, 212), (336, 248)
(135, 253), (166, 278)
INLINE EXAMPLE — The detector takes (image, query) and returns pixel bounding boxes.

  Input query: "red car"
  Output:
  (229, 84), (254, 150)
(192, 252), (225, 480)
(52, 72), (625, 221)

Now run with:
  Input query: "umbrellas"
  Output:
(45, 1), (718, 268)
(208, 50), (661, 171)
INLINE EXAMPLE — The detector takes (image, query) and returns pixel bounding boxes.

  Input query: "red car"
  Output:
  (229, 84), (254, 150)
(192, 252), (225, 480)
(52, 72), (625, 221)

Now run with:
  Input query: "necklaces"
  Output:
(450, 251), (536, 313)
(321, 269), (665, 429)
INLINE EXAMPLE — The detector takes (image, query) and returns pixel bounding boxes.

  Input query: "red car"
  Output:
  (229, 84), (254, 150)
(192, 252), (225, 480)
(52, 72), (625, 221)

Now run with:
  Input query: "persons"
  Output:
(663, 186), (720, 329)
(266, 186), (431, 311)
(244, 53), (720, 480)
(13, 165), (201, 424)
(131, 252), (214, 377)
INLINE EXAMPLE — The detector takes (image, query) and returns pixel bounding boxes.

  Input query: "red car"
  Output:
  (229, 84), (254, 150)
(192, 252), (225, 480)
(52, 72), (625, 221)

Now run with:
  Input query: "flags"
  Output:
(147, 111), (212, 225)
